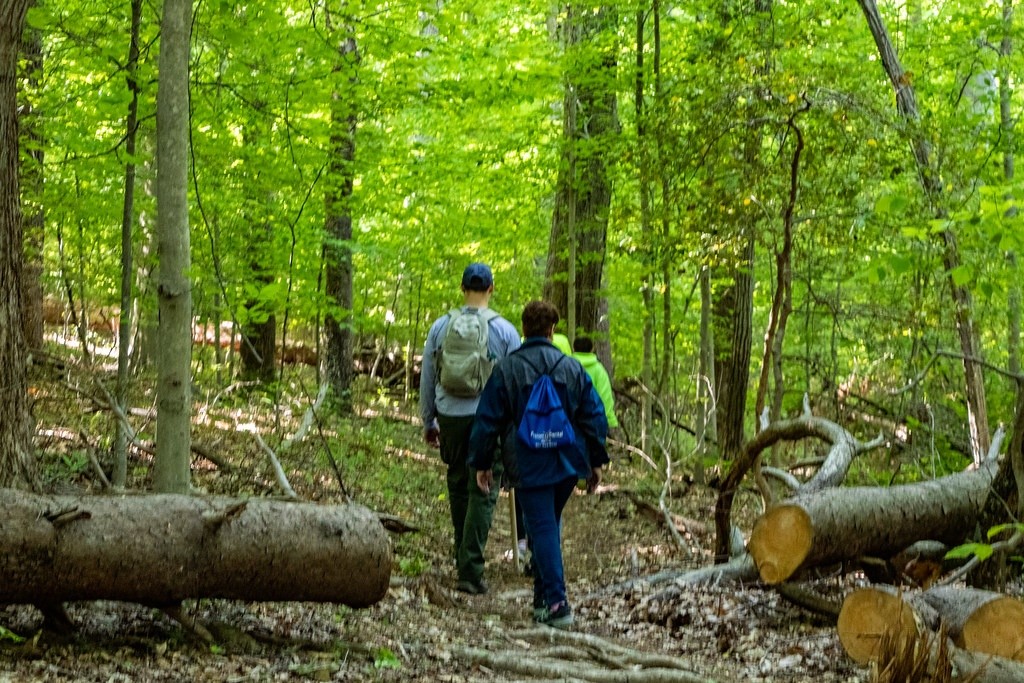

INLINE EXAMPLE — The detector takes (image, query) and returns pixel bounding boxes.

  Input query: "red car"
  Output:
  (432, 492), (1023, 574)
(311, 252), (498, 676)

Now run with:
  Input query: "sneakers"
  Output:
(532, 596), (576, 627)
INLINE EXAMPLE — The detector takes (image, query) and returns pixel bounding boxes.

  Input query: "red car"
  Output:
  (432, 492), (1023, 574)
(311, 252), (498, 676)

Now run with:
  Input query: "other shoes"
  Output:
(505, 547), (527, 564)
(454, 574), (492, 593)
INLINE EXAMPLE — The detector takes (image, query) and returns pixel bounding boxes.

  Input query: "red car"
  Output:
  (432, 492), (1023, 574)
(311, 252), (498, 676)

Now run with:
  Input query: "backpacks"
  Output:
(433, 306), (502, 397)
(513, 351), (577, 447)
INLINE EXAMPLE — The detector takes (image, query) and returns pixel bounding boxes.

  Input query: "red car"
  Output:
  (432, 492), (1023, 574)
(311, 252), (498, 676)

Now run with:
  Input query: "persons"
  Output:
(470, 301), (610, 627)
(419, 264), (522, 594)
(564, 335), (619, 426)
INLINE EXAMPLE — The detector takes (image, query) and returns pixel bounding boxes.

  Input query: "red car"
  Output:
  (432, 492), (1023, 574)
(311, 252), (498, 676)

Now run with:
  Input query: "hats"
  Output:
(462, 264), (491, 289)
